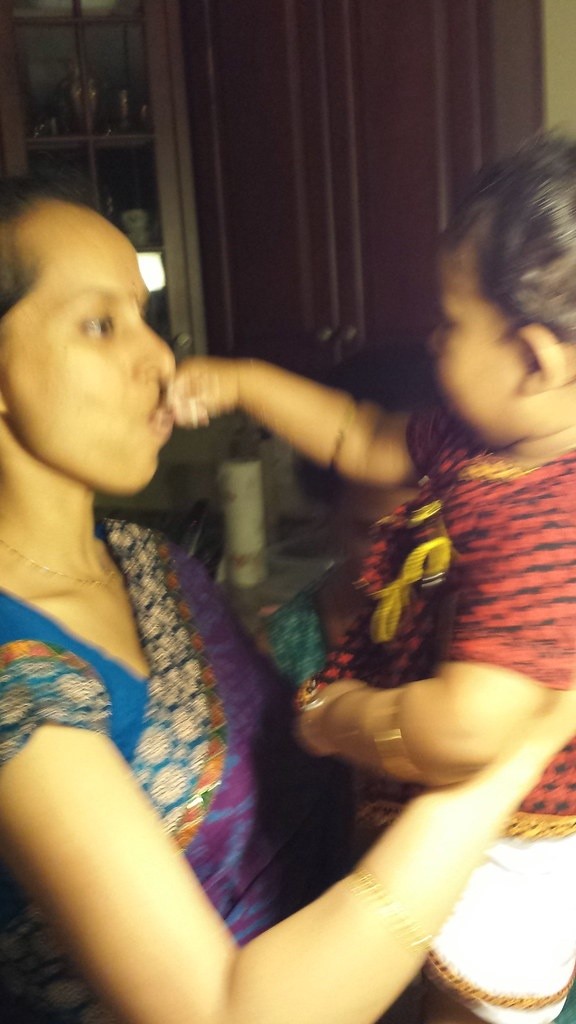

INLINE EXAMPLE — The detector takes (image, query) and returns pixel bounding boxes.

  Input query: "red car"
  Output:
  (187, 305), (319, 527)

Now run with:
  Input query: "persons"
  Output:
(0, 132), (576, 1024)
(160, 135), (576, 1024)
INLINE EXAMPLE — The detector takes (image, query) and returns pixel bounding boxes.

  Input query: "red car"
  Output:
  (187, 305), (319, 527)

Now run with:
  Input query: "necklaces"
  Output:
(0, 537), (120, 587)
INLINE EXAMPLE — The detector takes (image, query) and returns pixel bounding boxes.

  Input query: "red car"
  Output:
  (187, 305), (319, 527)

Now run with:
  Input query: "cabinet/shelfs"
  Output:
(182, 0), (543, 370)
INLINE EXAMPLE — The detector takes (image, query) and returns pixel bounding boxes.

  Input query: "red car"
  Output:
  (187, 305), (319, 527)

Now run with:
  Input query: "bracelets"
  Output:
(329, 398), (360, 470)
(373, 687), (425, 783)
